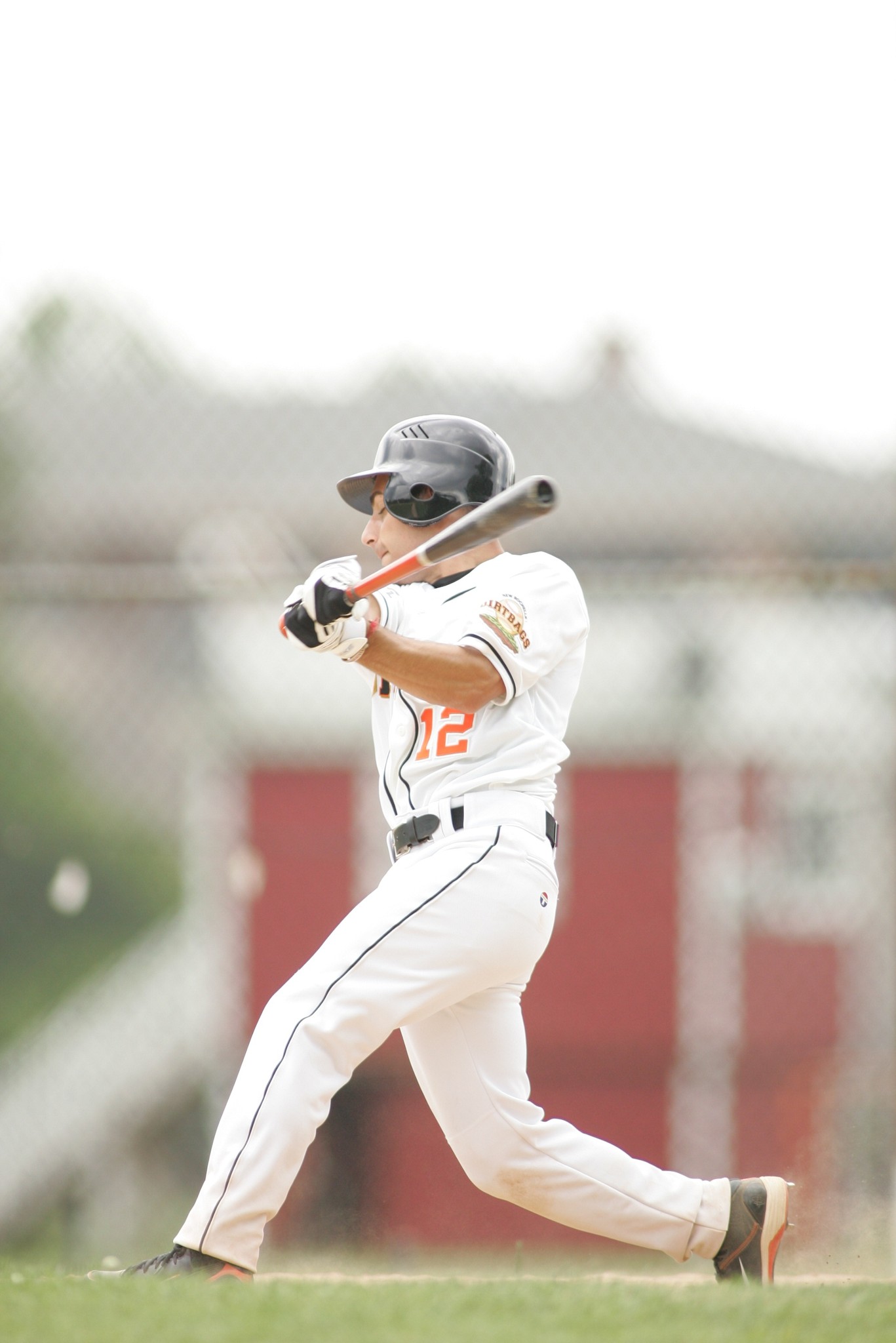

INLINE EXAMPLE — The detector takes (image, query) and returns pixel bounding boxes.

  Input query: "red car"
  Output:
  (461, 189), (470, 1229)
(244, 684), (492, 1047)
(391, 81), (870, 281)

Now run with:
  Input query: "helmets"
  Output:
(334, 415), (518, 524)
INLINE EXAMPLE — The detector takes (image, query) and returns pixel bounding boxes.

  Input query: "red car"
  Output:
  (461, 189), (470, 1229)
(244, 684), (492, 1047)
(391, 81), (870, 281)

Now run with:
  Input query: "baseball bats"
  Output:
(277, 474), (556, 640)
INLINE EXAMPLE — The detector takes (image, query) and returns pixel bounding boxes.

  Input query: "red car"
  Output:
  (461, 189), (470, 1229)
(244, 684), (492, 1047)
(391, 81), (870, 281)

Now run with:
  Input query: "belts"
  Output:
(384, 802), (560, 863)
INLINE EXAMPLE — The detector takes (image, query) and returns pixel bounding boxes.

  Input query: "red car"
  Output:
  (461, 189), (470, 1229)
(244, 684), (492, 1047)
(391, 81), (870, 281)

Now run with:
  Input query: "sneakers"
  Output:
(82, 1240), (256, 1282)
(709, 1177), (795, 1283)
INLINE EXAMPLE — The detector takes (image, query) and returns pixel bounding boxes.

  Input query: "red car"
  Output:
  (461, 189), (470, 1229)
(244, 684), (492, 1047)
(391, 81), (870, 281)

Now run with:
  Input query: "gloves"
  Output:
(300, 556), (366, 624)
(281, 580), (373, 664)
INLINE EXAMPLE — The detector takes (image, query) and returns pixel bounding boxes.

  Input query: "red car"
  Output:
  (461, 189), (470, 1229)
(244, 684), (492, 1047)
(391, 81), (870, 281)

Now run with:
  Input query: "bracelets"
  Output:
(366, 619), (379, 638)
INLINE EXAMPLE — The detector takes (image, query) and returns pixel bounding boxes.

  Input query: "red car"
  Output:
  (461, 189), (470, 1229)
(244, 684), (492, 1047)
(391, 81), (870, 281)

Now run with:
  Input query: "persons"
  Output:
(88, 415), (795, 1285)
